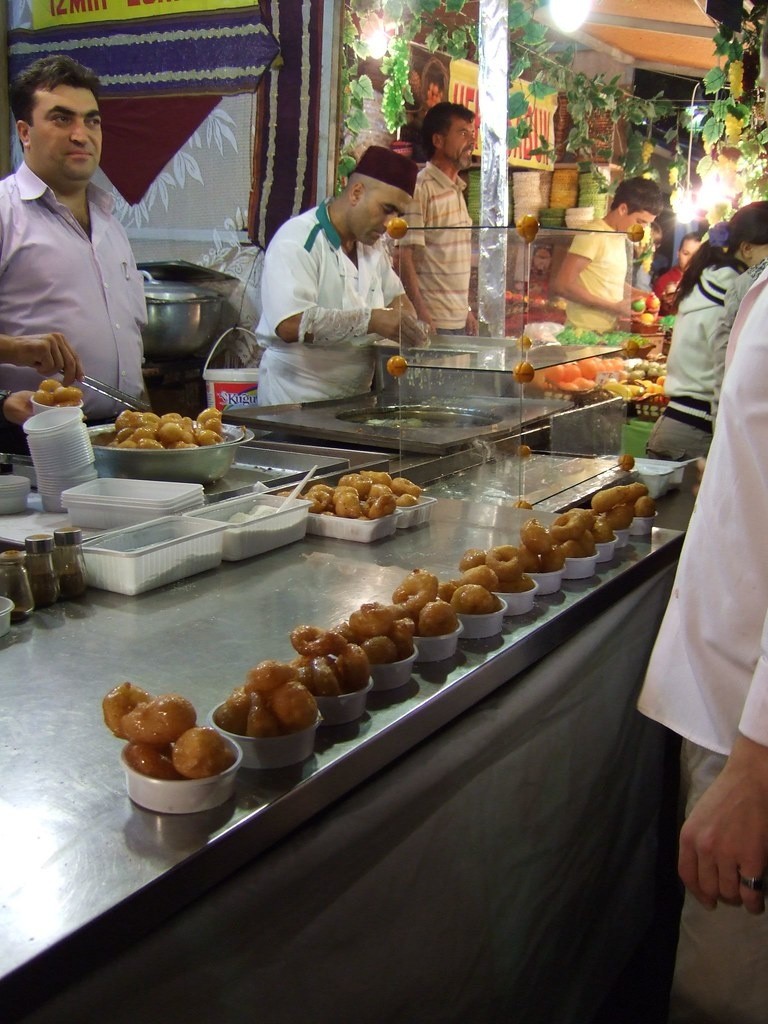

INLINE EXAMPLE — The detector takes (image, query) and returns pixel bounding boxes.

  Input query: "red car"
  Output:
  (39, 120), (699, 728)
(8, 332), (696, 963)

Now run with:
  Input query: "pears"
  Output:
(628, 362), (666, 381)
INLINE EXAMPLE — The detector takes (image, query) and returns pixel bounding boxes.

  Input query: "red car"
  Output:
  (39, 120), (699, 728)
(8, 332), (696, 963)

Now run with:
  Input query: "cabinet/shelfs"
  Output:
(335, 33), (634, 243)
(384, 220), (631, 512)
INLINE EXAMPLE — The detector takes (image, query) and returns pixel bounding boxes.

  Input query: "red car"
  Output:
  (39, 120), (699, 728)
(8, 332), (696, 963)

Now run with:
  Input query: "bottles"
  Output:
(24, 535), (61, 609)
(1, 550), (34, 624)
(53, 526), (90, 600)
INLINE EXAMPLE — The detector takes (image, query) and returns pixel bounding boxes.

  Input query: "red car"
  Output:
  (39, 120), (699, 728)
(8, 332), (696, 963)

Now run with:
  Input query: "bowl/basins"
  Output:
(613, 523), (633, 548)
(594, 533), (619, 563)
(468, 167), (610, 229)
(119, 733), (243, 814)
(315, 672), (374, 727)
(208, 699), (321, 770)
(524, 563), (566, 595)
(599, 339), (656, 361)
(630, 510), (658, 535)
(620, 319), (662, 334)
(597, 452), (688, 498)
(330, 643), (419, 691)
(456, 596), (508, 638)
(563, 550), (600, 579)
(491, 579), (539, 616)
(0, 396), (437, 637)
(412, 617), (465, 662)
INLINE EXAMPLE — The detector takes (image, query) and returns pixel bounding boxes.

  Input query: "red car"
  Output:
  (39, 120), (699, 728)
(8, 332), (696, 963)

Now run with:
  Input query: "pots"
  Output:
(142, 280), (225, 362)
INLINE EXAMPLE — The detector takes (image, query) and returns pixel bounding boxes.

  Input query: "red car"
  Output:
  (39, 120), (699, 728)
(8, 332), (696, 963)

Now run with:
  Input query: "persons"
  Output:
(638, 202), (768, 1024)
(391, 102), (477, 335)
(553, 177), (663, 333)
(631, 222), (662, 293)
(254, 145), (428, 407)
(0, 55), (149, 457)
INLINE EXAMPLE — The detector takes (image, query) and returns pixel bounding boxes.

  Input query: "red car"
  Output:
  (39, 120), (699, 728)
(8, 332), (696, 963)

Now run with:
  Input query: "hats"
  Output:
(353, 145), (418, 198)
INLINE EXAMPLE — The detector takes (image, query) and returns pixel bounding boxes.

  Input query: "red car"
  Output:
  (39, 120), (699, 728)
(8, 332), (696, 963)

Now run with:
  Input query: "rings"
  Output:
(741, 876), (765, 891)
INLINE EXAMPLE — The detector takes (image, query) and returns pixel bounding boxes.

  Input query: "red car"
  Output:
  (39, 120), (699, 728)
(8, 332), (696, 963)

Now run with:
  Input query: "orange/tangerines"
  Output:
(543, 357), (623, 390)
(635, 376), (666, 395)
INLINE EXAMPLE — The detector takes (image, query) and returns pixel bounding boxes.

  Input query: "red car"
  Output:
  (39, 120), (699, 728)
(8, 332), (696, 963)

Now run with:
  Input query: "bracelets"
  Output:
(468, 306), (472, 311)
(0, 389), (12, 412)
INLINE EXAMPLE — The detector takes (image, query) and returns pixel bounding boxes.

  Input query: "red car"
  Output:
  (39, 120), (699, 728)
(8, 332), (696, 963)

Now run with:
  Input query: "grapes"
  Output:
(729, 47), (758, 98)
(643, 112), (743, 229)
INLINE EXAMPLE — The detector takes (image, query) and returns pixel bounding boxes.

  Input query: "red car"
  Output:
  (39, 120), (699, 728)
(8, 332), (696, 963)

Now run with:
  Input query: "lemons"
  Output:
(633, 300), (654, 323)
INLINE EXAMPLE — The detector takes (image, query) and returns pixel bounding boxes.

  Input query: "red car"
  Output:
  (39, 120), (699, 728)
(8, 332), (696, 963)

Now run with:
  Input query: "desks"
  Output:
(0, 453), (720, 1024)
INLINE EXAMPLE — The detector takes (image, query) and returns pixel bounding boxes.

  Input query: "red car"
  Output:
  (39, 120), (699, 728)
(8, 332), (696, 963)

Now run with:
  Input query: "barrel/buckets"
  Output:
(203, 327), (262, 411)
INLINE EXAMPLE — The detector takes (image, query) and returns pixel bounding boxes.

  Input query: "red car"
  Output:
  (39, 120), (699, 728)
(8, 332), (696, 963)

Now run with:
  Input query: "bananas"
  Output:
(603, 382), (642, 398)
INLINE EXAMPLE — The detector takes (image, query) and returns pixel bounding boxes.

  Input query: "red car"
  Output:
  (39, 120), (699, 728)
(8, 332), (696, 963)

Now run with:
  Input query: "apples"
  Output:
(647, 296), (660, 308)
